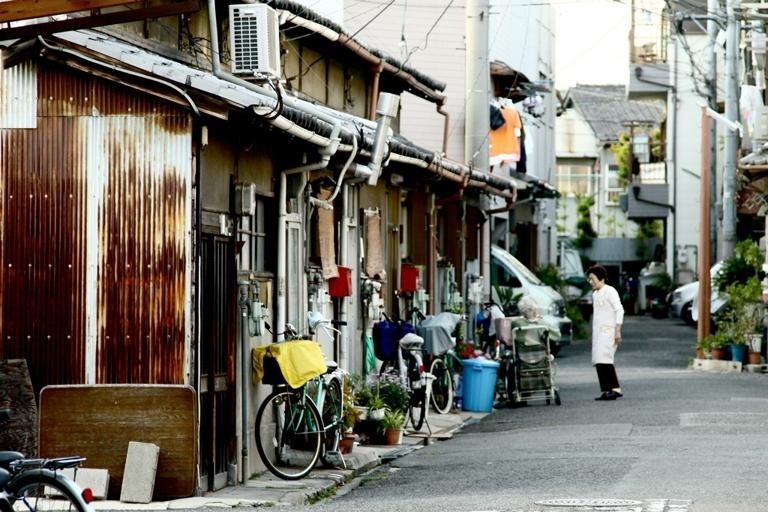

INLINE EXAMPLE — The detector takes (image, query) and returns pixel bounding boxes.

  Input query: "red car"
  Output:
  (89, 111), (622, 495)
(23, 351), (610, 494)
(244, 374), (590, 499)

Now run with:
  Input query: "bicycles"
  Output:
(251, 318), (356, 479)
(373, 290), (504, 434)
(0, 450), (90, 511)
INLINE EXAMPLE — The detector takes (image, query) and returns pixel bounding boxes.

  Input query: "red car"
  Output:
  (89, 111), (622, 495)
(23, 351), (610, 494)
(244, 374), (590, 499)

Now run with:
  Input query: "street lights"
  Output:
(631, 63), (677, 266)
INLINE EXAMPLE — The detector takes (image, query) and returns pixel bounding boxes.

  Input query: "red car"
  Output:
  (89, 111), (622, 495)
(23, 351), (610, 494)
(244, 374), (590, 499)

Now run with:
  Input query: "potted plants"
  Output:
(368, 380), (391, 420)
(352, 383), (374, 422)
(378, 408), (407, 445)
(692, 238), (768, 374)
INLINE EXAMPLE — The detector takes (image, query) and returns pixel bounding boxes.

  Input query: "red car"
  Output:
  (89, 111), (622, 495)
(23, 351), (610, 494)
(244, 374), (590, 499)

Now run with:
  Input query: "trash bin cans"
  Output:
(460, 356), (501, 412)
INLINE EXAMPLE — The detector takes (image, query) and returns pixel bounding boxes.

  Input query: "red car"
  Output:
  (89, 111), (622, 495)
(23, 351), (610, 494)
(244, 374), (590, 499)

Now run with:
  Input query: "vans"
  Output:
(491, 245), (572, 357)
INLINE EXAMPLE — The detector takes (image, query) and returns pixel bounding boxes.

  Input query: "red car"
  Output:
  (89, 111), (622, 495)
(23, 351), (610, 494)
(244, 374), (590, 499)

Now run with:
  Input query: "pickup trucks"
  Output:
(557, 242), (594, 307)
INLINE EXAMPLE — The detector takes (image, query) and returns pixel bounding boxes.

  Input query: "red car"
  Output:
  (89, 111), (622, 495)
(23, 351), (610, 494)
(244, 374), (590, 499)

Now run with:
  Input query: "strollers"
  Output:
(500, 325), (561, 406)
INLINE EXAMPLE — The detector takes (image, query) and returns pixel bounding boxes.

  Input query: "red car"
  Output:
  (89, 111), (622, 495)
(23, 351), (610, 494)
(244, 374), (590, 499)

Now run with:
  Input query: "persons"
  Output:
(585, 265), (627, 401)
(506, 297), (562, 349)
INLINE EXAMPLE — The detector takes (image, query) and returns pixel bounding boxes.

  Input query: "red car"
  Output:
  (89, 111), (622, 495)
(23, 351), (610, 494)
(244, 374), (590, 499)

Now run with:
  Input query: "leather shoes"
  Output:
(595, 388), (623, 401)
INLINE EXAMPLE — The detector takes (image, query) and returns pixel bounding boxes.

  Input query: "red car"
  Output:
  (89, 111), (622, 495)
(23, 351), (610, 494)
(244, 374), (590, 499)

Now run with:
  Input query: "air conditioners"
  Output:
(229, 3), (281, 81)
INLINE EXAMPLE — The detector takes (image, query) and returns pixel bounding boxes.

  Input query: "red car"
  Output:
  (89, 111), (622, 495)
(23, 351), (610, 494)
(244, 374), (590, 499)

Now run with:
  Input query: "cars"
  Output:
(667, 260), (768, 335)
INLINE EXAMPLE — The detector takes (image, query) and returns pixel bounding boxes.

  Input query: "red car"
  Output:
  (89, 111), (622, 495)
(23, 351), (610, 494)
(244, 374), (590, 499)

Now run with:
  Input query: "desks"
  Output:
(37, 384), (198, 500)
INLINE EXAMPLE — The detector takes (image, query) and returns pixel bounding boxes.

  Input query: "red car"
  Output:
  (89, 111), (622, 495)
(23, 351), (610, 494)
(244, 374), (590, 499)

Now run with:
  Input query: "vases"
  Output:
(339, 436), (355, 454)
(369, 423), (383, 445)
